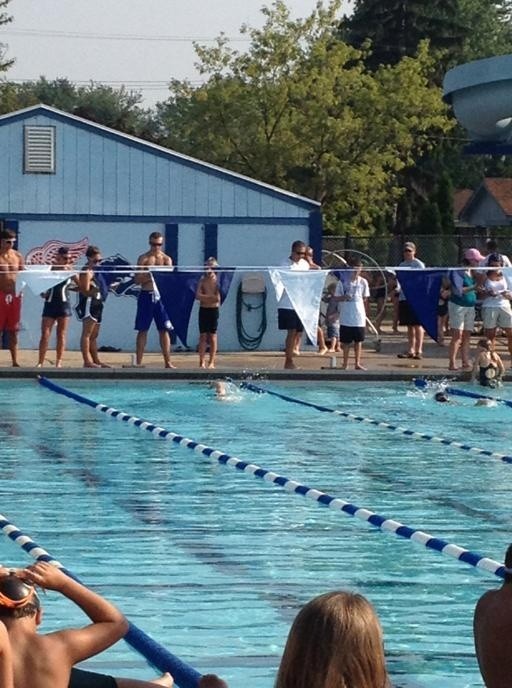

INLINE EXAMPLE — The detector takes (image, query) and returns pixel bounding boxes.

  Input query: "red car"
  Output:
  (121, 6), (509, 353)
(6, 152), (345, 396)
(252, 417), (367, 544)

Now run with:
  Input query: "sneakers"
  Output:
(397, 352), (416, 358)
(414, 352), (423, 358)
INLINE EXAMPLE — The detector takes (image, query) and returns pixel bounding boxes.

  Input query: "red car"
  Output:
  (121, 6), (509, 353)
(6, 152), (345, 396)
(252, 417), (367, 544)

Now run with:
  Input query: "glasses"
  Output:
(295, 250), (305, 255)
(149, 240), (163, 246)
(87, 255), (102, 263)
(60, 254), (73, 260)
(3, 239), (17, 244)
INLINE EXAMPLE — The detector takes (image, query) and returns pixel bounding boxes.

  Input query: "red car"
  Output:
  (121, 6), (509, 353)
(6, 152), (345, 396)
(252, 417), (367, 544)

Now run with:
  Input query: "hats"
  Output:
(0, 568), (34, 609)
(403, 242), (416, 251)
(464, 248), (486, 261)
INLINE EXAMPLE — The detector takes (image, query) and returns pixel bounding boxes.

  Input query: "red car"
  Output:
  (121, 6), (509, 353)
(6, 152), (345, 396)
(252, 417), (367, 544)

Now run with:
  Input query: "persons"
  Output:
(195, 257), (221, 370)
(0, 560), (174, 688)
(398, 241), (426, 359)
(34, 247), (80, 369)
(276, 591), (391, 687)
(79, 246), (114, 368)
(0, 228), (25, 368)
(207, 377), (230, 401)
(438, 240), (512, 370)
(133, 233), (179, 369)
(376, 271), (399, 333)
(474, 545), (511, 687)
(277, 241), (369, 372)
(471, 339), (505, 387)
